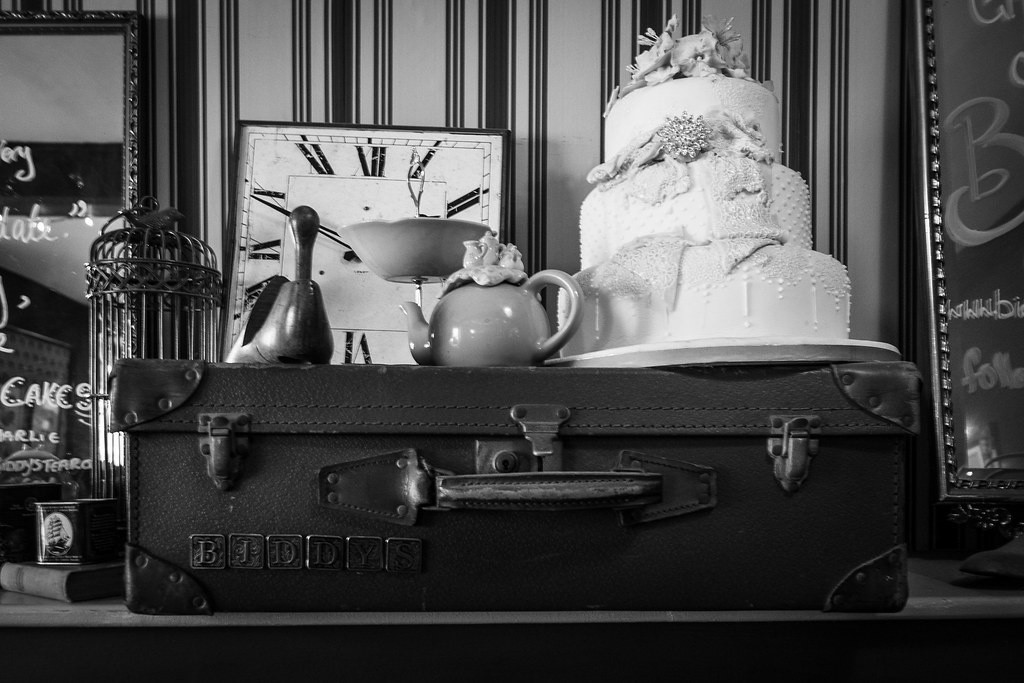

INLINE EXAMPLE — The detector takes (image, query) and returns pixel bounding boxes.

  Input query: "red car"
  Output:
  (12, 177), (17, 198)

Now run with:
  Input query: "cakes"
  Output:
(555, 74), (852, 355)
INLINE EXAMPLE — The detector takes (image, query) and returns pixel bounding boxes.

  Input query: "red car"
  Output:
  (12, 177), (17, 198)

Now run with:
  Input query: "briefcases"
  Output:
(108, 355), (919, 616)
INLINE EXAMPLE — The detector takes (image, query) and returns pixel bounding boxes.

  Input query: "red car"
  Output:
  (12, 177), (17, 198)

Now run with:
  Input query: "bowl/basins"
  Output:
(336, 217), (491, 282)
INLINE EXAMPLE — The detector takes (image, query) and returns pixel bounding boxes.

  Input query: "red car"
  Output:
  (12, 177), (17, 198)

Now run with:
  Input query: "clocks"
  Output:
(217, 120), (513, 364)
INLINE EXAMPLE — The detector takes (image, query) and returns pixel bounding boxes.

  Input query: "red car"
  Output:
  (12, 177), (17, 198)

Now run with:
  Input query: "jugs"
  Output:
(401, 232), (584, 368)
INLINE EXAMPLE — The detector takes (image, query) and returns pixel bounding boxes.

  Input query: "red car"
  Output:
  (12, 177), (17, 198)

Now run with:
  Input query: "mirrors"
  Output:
(898, 2), (1023, 545)
(0, 9), (150, 567)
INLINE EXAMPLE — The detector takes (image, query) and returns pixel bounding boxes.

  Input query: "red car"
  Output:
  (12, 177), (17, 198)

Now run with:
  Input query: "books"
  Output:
(0, 562), (127, 604)
